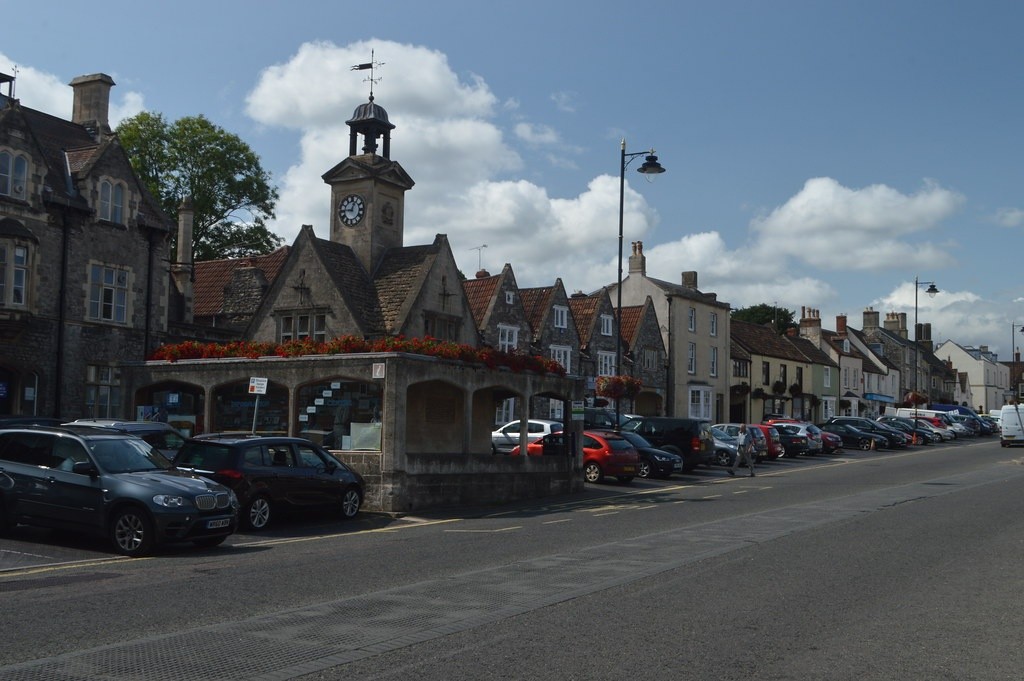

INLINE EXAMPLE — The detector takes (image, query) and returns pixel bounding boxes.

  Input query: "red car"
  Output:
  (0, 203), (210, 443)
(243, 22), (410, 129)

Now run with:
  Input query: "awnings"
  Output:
(864, 392), (894, 404)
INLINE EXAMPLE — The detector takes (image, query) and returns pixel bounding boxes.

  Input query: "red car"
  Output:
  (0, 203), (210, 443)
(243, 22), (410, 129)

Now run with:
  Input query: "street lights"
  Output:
(914, 276), (940, 430)
(1011, 322), (1024, 396)
(614, 137), (667, 424)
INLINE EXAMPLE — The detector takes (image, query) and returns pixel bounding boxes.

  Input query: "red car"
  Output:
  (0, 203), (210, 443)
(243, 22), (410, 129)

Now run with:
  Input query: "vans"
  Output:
(618, 418), (717, 473)
(1000, 403), (1024, 447)
(896, 403), (990, 438)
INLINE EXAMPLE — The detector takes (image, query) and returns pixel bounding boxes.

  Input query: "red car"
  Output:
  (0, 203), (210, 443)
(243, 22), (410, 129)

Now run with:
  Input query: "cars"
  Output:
(710, 418), (843, 466)
(979, 414), (1000, 434)
(821, 416), (954, 451)
(492, 417), (682, 484)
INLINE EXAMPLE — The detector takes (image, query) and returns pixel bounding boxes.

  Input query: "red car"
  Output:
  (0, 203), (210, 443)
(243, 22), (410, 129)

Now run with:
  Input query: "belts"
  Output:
(739, 445), (744, 447)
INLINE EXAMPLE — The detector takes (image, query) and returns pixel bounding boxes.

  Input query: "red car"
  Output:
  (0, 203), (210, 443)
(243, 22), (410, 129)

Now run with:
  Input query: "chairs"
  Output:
(273, 450), (288, 467)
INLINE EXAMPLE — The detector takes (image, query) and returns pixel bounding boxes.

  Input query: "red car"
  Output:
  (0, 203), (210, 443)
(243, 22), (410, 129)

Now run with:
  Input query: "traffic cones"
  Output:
(911, 432), (917, 446)
(870, 439), (876, 451)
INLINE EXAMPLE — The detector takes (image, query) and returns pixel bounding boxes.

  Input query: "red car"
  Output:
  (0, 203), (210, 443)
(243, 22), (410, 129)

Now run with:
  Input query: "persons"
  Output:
(727, 424), (756, 477)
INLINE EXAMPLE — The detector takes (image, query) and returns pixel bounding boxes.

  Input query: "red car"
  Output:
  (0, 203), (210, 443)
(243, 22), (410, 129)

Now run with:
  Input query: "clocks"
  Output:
(337, 194), (366, 228)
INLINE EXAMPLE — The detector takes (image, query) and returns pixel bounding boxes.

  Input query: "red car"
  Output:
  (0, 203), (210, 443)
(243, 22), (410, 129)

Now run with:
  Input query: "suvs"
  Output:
(0, 416), (369, 557)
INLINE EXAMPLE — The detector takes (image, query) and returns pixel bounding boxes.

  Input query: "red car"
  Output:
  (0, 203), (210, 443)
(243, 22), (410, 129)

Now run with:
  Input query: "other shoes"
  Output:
(727, 469), (734, 475)
(750, 473), (755, 477)
